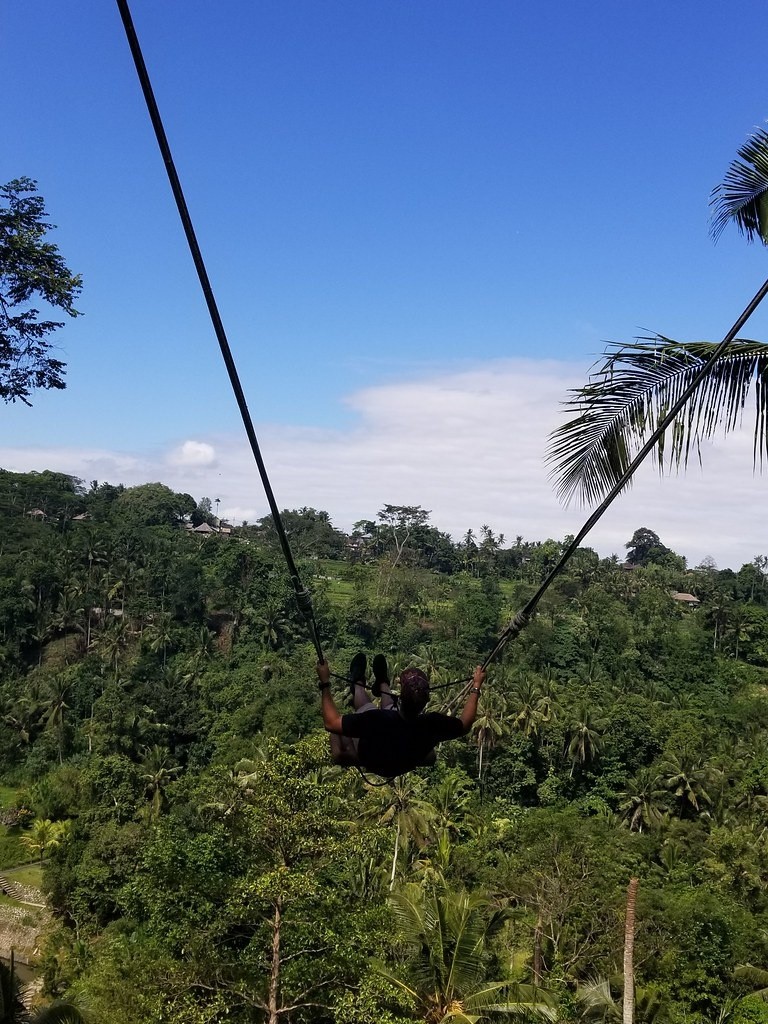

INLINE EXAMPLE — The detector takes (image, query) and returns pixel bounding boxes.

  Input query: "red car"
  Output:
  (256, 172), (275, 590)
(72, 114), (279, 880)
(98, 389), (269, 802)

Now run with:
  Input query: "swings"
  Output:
(112, 0), (767, 778)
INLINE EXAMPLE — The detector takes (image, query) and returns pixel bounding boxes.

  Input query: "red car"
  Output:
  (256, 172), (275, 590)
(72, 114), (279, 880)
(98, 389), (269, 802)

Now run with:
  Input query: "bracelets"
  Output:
(319, 682), (332, 690)
(471, 687), (480, 694)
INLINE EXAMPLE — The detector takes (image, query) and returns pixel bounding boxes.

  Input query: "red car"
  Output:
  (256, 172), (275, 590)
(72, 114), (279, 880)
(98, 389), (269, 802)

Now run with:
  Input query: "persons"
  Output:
(316, 653), (486, 777)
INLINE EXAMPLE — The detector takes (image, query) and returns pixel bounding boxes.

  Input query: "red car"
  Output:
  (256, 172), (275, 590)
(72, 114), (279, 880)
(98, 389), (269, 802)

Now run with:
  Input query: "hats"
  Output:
(400, 668), (430, 707)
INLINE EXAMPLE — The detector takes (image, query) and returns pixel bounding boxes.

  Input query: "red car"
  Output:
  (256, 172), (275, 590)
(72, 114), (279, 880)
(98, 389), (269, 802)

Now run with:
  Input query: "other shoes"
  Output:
(372, 654), (391, 697)
(350, 653), (367, 697)
